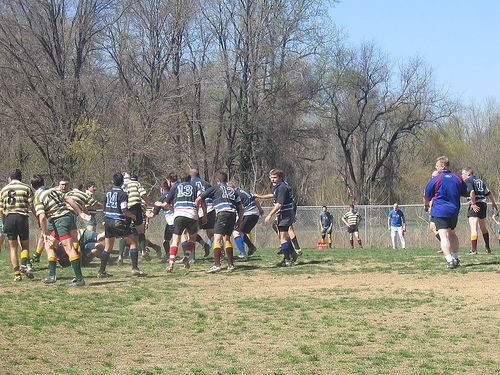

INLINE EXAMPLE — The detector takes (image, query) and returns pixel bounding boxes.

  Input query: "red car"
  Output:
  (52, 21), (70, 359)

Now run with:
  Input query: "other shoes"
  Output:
(141, 250), (151, 261)
(465, 249), (477, 254)
(117, 255), (123, 264)
(219, 245), (225, 256)
(204, 243), (210, 257)
(485, 249), (491, 255)
(154, 245), (161, 259)
(161, 253), (170, 260)
(446, 258), (460, 268)
(109, 256), (118, 267)
(174, 256), (196, 263)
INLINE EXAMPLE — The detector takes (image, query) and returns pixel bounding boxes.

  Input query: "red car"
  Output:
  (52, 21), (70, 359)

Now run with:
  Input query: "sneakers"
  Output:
(131, 269), (147, 276)
(26, 263), (35, 272)
(290, 253), (298, 263)
(294, 249), (303, 255)
(205, 265), (221, 273)
(65, 277), (84, 286)
(227, 264), (236, 270)
(277, 249), (284, 254)
(42, 275), (56, 283)
(234, 254), (247, 261)
(248, 247), (256, 257)
(183, 257), (190, 269)
(19, 265), (34, 279)
(166, 265), (173, 272)
(14, 275), (21, 281)
(97, 272), (113, 278)
(29, 253), (40, 263)
(277, 258), (291, 266)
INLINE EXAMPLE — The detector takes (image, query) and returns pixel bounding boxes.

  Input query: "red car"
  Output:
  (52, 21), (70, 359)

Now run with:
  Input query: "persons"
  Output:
(28, 174), (88, 286)
(423, 156), (467, 268)
(195, 178), (236, 272)
(47, 184), (105, 253)
(265, 170), (298, 267)
(492, 209), (500, 251)
(97, 173), (145, 278)
(388, 203), (406, 248)
(119, 175), (151, 264)
(58, 244), (115, 267)
(164, 173), (196, 270)
(148, 174), (208, 273)
(187, 167), (217, 257)
(461, 167), (498, 254)
(226, 182), (264, 261)
(253, 173), (303, 256)
(30, 177), (72, 262)
(145, 182), (183, 262)
(0, 169), (40, 281)
(341, 205), (363, 248)
(424, 171), (443, 253)
(84, 180), (104, 231)
(319, 206), (333, 248)
(53, 228), (121, 252)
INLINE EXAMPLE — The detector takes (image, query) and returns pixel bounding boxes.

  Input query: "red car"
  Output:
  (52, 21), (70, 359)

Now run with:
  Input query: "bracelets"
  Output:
(424, 202), (428, 206)
